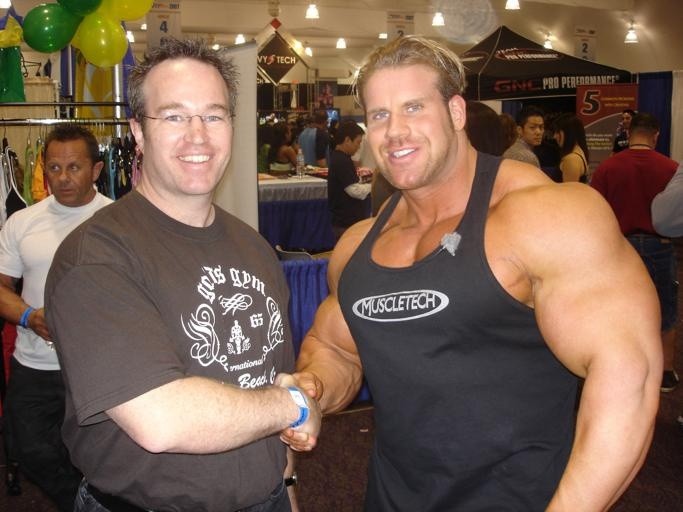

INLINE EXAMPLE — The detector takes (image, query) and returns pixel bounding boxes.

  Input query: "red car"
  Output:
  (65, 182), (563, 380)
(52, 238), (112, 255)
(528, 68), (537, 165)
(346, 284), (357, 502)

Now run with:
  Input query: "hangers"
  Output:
(0, 118), (19, 168)
(26, 117), (128, 166)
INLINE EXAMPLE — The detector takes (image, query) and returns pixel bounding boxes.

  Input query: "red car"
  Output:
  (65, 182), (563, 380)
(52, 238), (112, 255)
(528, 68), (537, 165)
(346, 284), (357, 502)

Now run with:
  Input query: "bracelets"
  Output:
(19, 305), (35, 330)
(286, 386), (310, 428)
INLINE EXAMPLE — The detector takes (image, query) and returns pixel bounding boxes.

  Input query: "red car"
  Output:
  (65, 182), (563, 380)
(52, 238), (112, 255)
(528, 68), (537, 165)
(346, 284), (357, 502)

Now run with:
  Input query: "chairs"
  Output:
(275, 245), (311, 261)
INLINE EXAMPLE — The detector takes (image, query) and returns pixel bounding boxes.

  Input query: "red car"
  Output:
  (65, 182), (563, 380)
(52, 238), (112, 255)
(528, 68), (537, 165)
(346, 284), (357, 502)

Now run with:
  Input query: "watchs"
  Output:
(285, 474), (298, 486)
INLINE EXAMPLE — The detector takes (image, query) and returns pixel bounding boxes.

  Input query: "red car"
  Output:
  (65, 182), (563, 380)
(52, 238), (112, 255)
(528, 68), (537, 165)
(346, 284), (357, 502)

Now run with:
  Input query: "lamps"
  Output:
(336, 37), (347, 50)
(624, 19), (639, 43)
(505, 1), (521, 10)
(543, 33), (552, 50)
(431, 8), (445, 27)
(305, 1), (319, 19)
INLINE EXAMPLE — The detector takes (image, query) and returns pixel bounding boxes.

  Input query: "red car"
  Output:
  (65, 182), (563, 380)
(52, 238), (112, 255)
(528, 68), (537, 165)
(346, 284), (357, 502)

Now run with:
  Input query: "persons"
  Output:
(587, 111), (680, 392)
(650, 158), (683, 238)
(44, 39), (298, 511)
(275, 35), (664, 512)
(614, 112), (635, 152)
(254, 98), (589, 243)
(0, 124), (117, 509)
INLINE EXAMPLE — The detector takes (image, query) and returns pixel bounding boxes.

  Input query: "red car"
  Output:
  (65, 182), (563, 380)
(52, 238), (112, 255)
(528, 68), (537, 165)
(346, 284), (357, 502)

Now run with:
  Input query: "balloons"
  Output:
(23, 1), (154, 68)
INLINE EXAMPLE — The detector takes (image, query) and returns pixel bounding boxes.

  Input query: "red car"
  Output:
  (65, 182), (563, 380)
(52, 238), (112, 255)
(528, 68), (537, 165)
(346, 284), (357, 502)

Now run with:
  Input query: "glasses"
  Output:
(140, 108), (236, 128)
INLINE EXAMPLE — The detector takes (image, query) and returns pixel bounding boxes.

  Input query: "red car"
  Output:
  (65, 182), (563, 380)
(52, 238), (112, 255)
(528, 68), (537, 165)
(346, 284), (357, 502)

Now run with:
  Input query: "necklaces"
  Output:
(628, 144), (652, 149)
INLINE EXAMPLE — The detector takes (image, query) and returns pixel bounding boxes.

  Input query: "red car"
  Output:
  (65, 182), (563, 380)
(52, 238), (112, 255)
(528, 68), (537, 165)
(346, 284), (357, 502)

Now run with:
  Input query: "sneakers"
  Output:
(660, 369), (680, 393)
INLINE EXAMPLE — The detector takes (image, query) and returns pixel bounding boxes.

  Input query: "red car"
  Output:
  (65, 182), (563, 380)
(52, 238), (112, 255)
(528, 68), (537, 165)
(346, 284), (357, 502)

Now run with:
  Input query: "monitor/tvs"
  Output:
(314, 108), (340, 129)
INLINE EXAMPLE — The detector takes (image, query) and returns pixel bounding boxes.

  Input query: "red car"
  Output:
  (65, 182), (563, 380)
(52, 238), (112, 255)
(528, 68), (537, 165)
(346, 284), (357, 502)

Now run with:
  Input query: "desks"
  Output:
(258, 175), (329, 252)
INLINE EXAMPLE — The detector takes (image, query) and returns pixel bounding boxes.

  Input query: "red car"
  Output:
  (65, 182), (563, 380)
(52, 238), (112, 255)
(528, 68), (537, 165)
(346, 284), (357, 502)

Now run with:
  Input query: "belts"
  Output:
(85, 482), (151, 512)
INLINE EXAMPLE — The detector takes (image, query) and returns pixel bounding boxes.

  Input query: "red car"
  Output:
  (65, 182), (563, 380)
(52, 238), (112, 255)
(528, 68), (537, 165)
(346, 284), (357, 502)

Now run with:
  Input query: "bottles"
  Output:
(296, 148), (305, 181)
